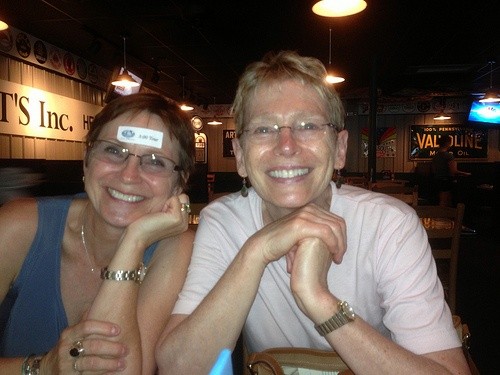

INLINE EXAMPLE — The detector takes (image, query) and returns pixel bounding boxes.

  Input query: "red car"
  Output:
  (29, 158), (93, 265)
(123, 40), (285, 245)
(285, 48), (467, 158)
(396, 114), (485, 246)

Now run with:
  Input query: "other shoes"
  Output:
(459, 226), (480, 235)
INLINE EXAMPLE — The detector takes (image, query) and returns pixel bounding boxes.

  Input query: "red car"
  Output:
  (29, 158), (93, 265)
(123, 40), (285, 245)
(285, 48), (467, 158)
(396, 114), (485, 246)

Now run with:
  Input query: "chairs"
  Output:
(412, 204), (461, 316)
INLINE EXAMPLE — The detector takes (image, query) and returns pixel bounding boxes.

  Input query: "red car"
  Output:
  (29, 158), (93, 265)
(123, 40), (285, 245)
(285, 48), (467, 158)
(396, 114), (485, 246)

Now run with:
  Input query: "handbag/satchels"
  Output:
(247, 346), (355, 374)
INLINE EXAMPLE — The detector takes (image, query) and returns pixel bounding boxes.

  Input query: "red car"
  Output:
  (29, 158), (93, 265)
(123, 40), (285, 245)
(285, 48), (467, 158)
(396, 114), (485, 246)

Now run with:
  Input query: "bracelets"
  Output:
(23, 353), (42, 375)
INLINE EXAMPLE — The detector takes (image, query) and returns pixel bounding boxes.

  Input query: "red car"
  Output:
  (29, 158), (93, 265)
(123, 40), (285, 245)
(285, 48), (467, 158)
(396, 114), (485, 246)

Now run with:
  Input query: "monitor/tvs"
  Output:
(465, 100), (500, 128)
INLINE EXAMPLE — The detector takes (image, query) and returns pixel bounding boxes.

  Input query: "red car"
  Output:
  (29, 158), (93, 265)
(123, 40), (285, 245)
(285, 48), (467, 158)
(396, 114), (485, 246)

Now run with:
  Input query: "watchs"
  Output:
(100, 262), (147, 284)
(314, 301), (356, 336)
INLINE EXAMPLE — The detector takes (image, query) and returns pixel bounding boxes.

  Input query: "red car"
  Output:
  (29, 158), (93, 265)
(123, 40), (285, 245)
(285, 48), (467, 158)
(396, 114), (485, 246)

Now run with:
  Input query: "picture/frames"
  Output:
(223, 130), (236, 157)
(409, 124), (489, 160)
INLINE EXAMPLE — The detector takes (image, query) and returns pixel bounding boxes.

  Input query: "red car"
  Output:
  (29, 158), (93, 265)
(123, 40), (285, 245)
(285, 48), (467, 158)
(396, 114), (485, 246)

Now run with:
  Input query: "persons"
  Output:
(430, 134), (464, 216)
(155, 51), (471, 375)
(0, 93), (195, 375)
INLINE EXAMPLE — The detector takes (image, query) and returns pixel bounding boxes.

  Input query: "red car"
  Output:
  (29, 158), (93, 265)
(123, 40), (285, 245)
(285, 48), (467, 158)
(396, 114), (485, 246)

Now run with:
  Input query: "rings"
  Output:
(181, 203), (191, 213)
(70, 340), (84, 356)
(73, 357), (83, 371)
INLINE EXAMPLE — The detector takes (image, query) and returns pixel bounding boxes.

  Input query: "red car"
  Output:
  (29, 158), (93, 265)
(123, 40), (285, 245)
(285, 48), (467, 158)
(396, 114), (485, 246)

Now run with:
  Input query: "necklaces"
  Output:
(80, 203), (100, 272)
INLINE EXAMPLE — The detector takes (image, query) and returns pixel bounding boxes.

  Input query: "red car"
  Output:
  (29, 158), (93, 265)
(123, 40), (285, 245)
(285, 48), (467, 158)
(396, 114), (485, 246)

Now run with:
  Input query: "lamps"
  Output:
(111, 37), (140, 87)
(0, 15), (9, 31)
(324, 28), (346, 84)
(312, 0), (367, 17)
(207, 97), (222, 125)
(176, 77), (194, 110)
(432, 97), (451, 121)
(479, 61), (500, 103)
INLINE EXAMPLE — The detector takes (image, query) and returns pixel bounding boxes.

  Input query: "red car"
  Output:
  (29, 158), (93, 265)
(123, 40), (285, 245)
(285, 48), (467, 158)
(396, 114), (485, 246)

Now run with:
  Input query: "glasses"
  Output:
(243, 120), (342, 145)
(93, 139), (183, 177)
